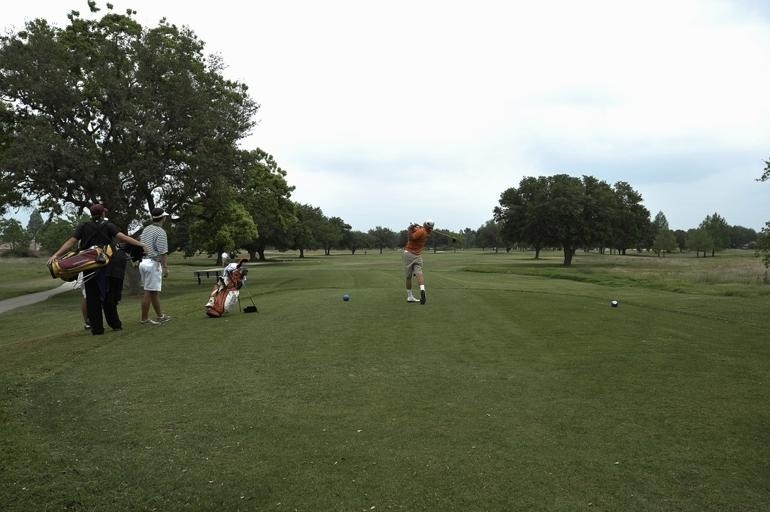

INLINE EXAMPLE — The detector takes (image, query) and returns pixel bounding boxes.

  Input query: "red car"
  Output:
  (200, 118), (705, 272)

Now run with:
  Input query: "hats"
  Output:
(153, 210), (169, 219)
(426, 220), (435, 230)
(90, 204), (108, 215)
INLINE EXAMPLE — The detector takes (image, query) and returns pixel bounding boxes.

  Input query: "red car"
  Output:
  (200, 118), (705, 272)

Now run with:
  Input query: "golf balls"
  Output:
(344, 295), (349, 301)
(611, 300), (617, 306)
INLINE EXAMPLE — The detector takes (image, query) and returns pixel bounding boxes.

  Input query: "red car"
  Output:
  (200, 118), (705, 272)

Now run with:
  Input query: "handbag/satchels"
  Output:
(205, 262), (248, 317)
(48, 244), (114, 282)
(125, 238), (145, 267)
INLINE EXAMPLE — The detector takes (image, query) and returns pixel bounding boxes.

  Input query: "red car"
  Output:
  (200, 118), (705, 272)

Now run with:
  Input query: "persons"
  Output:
(404, 220), (435, 304)
(46, 203), (148, 335)
(138, 207), (172, 326)
(78, 233), (92, 330)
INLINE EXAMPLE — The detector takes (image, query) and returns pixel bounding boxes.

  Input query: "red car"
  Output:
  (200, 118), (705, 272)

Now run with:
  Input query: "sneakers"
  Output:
(84, 322), (91, 328)
(141, 319), (161, 326)
(156, 313), (171, 322)
(114, 326), (122, 330)
(421, 290), (426, 304)
(407, 297), (420, 302)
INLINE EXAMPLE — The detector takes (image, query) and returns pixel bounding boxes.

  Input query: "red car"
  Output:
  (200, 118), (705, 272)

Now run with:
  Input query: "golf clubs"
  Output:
(411, 224), (457, 243)
(221, 252), (258, 312)
(120, 242), (146, 262)
